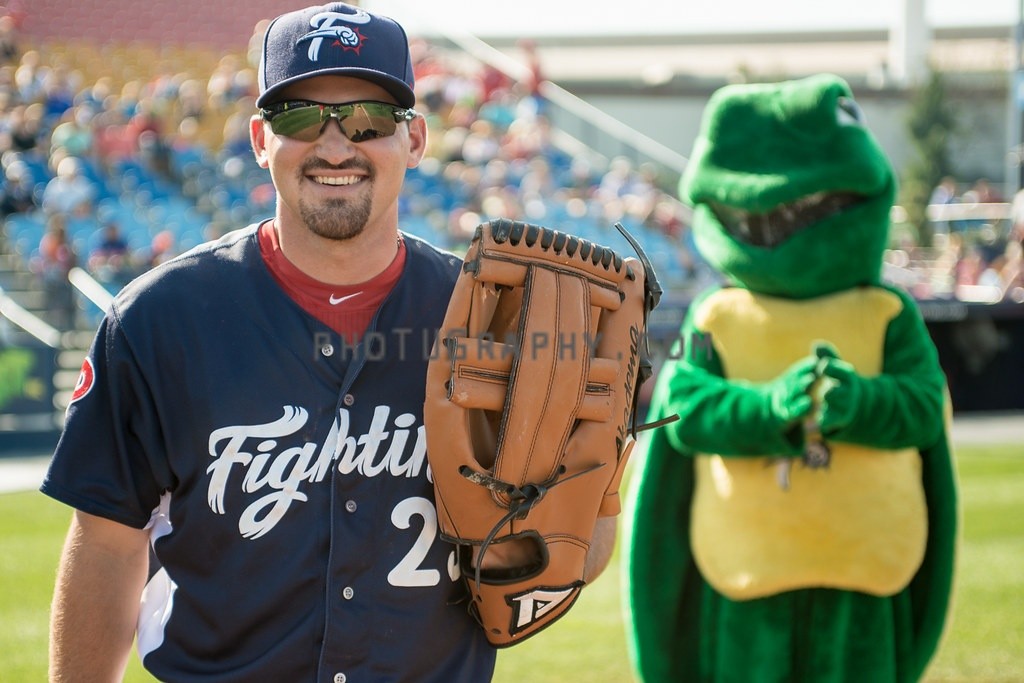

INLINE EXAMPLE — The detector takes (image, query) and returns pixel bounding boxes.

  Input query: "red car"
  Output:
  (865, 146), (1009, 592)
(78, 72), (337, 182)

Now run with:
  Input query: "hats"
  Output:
(255, 2), (415, 108)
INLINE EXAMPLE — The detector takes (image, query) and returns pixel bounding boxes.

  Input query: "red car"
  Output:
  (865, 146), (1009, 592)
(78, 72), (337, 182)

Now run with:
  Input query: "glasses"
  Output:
(263, 99), (417, 143)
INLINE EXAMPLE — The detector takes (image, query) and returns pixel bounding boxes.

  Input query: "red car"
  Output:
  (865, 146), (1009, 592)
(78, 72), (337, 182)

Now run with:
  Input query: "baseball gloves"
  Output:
(418, 216), (683, 654)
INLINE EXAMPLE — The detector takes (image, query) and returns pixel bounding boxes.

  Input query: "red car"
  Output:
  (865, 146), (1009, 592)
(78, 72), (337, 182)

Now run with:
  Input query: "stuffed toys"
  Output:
(624, 71), (958, 683)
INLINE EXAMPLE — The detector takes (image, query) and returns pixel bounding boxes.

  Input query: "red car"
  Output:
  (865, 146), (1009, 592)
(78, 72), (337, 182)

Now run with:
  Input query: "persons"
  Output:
(0, 15), (1023, 333)
(39, 1), (680, 683)
